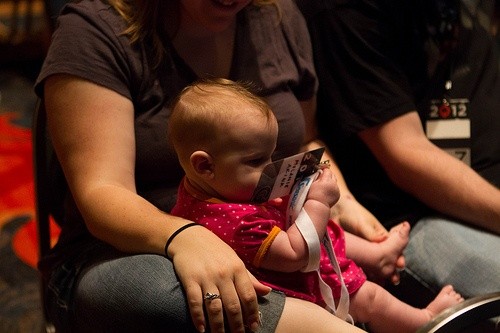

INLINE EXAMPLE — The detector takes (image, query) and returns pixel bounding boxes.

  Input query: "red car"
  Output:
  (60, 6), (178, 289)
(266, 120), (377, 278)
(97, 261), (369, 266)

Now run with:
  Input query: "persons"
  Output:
(168, 75), (466, 333)
(300, 0), (500, 298)
(31, 0), (500, 333)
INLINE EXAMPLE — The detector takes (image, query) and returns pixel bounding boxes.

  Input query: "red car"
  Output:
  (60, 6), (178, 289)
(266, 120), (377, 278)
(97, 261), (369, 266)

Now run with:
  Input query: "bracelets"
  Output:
(162, 222), (203, 256)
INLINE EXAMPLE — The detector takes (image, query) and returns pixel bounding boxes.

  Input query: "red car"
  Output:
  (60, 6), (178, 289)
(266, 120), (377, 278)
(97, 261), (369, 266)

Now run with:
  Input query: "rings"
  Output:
(203, 291), (220, 300)
